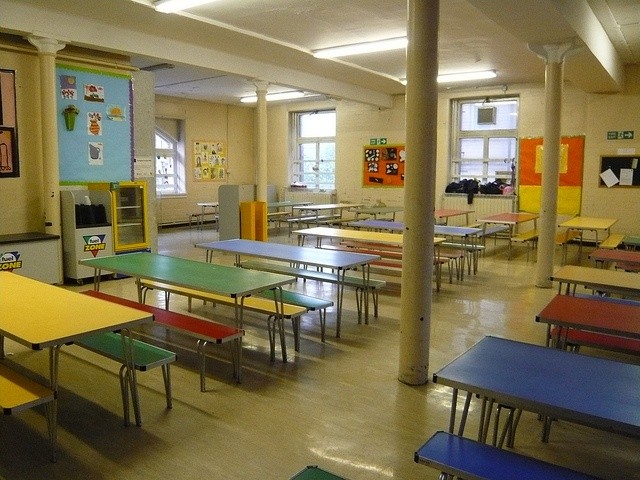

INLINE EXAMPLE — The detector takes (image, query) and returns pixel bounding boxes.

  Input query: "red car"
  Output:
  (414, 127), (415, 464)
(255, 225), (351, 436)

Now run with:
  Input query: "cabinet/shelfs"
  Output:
(91, 180), (151, 254)
(0, 231), (62, 285)
(59, 189), (113, 285)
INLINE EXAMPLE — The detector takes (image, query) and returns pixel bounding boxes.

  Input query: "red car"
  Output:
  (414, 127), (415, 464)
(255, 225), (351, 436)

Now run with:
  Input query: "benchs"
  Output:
(412, 430), (596, 479)
(555, 231), (581, 266)
(234, 259), (386, 326)
(267, 211), (292, 217)
(476, 223), (509, 258)
(614, 264), (640, 272)
(186, 211), (217, 229)
(370, 258), (403, 269)
(134, 277), (305, 363)
(457, 223), (481, 230)
(341, 240), (460, 286)
(623, 235), (640, 249)
(441, 241), (483, 273)
(0, 362), (54, 462)
(253, 287), (334, 345)
(511, 228), (539, 264)
(72, 331), (178, 428)
(599, 233), (624, 249)
(549, 325), (640, 362)
(78, 288), (245, 393)
(565, 289), (640, 306)
(316, 215), (368, 226)
(317, 244), (450, 293)
(286, 215), (343, 237)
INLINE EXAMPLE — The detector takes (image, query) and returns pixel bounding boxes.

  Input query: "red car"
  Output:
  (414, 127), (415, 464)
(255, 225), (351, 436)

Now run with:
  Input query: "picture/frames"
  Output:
(0, 127), (20, 179)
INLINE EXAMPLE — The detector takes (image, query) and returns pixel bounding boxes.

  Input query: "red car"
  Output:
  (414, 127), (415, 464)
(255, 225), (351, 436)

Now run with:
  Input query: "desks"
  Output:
(78, 250), (297, 382)
(550, 264), (640, 300)
(291, 226), (446, 295)
(557, 215), (619, 266)
(267, 201), (312, 228)
(435, 208), (474, 228)
(476, 210), (540, 259)
(292, 204), (364, 230)
(345, 218), (483, 281)
(535, 293), (640, 361)
(197, 201), (219, 232)
(432, 335), (640, 480)
(349, 206), (404, 231)
(588, 246), (640, 273)
(195, 239), (381, 338)
(1, 270), (156, 462)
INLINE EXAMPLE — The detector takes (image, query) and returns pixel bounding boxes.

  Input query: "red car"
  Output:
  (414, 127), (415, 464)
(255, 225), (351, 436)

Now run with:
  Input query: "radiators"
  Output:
(284, 189), (333, 222)
(441, 194), (514, 229)
